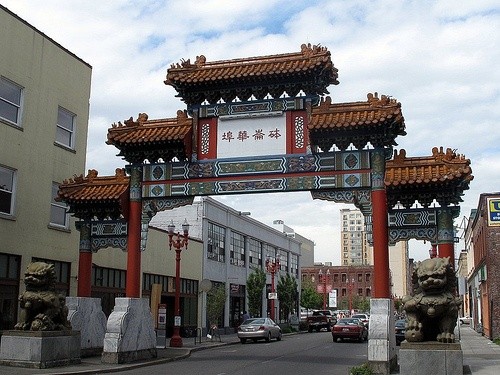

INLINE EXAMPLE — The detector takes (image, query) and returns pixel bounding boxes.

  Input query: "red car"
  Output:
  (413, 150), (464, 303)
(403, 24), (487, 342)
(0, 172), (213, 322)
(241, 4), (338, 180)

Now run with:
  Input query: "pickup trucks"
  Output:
(306, 310), (337, 333)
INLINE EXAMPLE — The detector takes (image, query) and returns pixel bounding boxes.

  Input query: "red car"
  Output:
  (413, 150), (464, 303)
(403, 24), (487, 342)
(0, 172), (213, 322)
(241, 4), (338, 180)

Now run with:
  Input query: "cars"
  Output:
(394, 320), (406, 340)
(336, 308), (369, 324)
(332, 318), (368, 343)
(237, 317), (282, 343)
(459, 316), (472, 326)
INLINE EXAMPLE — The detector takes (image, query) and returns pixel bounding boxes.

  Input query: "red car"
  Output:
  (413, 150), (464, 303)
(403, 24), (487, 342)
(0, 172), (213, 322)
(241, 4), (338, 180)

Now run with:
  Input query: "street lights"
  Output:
(346, 278), (355, 314)
(167, 218), (189, 347)
(268, 253), (279, 321)
(319, 266), (331, 310)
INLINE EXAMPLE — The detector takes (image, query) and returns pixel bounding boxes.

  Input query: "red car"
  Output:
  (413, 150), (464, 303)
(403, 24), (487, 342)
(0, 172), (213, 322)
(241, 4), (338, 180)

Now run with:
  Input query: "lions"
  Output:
(14, 261), (74, 330)
(403, 255), (464, 343)
